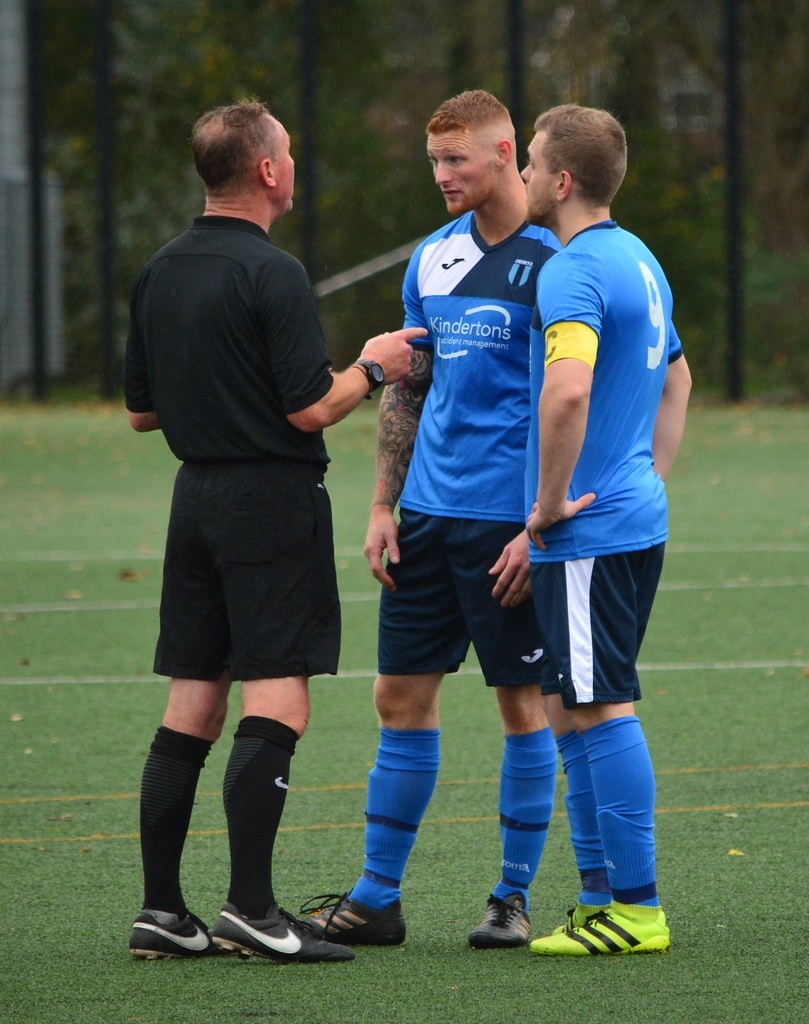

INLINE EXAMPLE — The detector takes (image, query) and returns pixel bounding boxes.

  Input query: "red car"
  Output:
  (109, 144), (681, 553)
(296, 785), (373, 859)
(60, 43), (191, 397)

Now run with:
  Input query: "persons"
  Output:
(121, 91), (429, 967)
(520, 101), (695, 956)
(295, 88), (575, 950)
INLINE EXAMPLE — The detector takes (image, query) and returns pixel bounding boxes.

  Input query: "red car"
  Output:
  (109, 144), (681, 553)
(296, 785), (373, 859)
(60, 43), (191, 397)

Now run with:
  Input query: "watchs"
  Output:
(349, 356), (385, 404)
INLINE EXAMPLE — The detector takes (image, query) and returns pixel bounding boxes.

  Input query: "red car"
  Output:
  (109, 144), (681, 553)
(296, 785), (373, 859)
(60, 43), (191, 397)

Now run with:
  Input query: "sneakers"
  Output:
(552, 914), (582, 935)
(530, 908), (670, 954)
(211, 901), (355, 963)
(127, 911), (223, 959)
(469, 899), (531, 947)
(296, 898), (407, 946)
